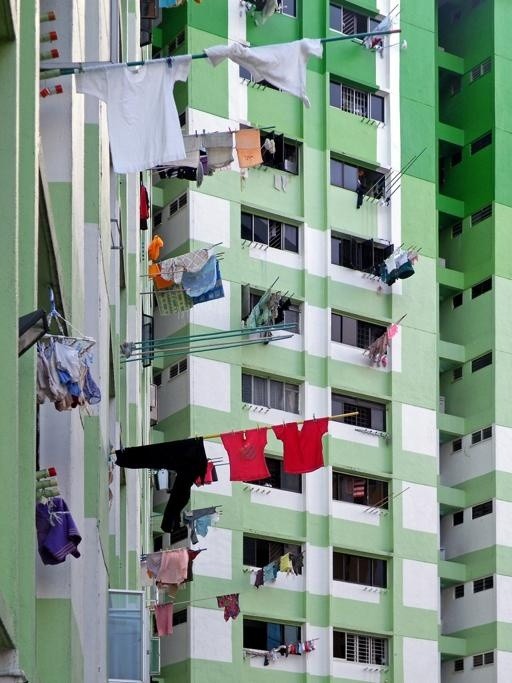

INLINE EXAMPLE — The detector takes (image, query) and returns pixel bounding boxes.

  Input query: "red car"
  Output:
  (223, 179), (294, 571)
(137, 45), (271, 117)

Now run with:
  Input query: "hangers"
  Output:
(37, 284), (96, 354)
